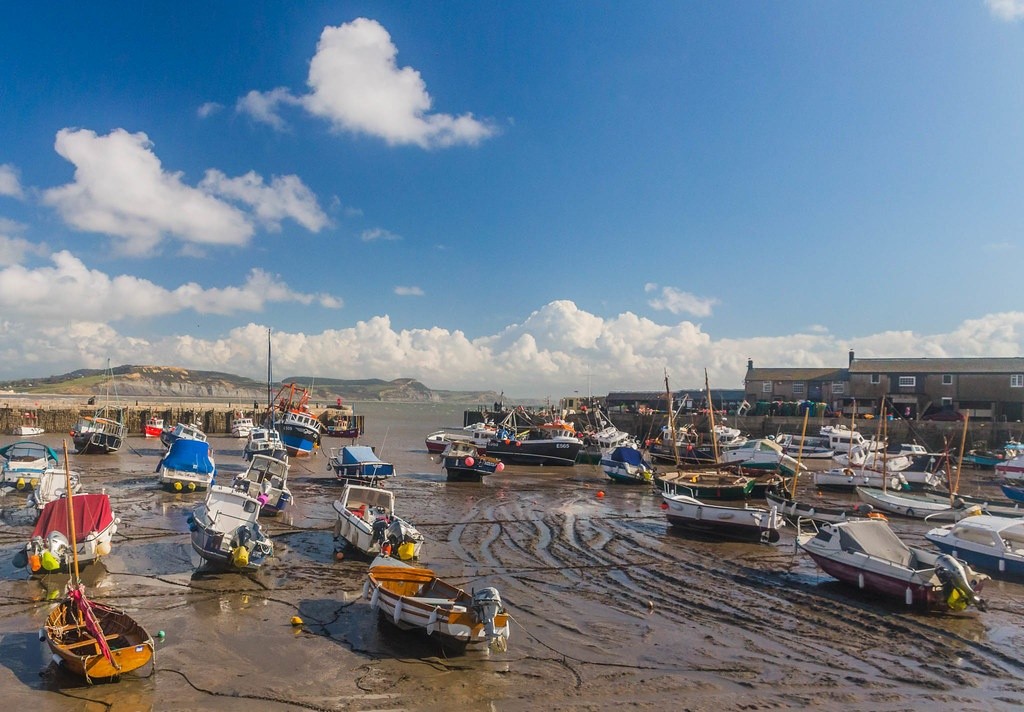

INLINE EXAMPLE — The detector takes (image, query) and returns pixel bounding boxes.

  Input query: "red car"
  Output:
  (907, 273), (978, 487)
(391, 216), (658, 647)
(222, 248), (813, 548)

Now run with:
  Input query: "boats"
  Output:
(144, 416), (215, 491)
(424, 366), (888, 485)
(327, 426), (359, 438)
(795, 515), (991, 611)
(12, 426), (44, 435)
(186, 453), (293, 572)
(25, 468), (121, 576)
(326, 443), (396, 486)
(962, 438), (1024, 503)
(855, 408), (989, 522)
(0, 441), (59, 491)
(362, 551), (512, 655)
(661, 479), (786, 538)
(232, 328), (322, 462)
(332, 483), (424, 558)
(923, 511), (1024, 576)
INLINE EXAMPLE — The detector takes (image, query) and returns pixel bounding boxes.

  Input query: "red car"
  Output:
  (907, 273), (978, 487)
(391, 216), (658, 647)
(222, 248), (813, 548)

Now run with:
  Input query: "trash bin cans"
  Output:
(136, 400), (139, 406)
(253, 403), (258, 409)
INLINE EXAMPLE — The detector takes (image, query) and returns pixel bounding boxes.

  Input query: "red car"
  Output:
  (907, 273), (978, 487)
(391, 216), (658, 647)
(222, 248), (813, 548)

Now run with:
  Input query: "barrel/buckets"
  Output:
(233, 546), (248, 567)
(16, 478), (25, 490)
(398, 543), (415, 559)
(381, 541), (391, 555)
(42, 552), (60, 570)
(28, 555), (42, 571)
(948, 589), (968, 611)
(257, 495), (268, 505)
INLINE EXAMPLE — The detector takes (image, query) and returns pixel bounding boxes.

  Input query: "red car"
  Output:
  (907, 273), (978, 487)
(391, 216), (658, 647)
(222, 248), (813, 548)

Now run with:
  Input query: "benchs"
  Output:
(52, 617), (131, 649)
(405, 596), (465, 604)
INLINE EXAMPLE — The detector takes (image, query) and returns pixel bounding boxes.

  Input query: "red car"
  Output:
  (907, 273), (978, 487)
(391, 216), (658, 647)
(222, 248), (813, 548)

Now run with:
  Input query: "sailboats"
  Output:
(70, 357), (129, 454)
(39, 438), (156, 684)
